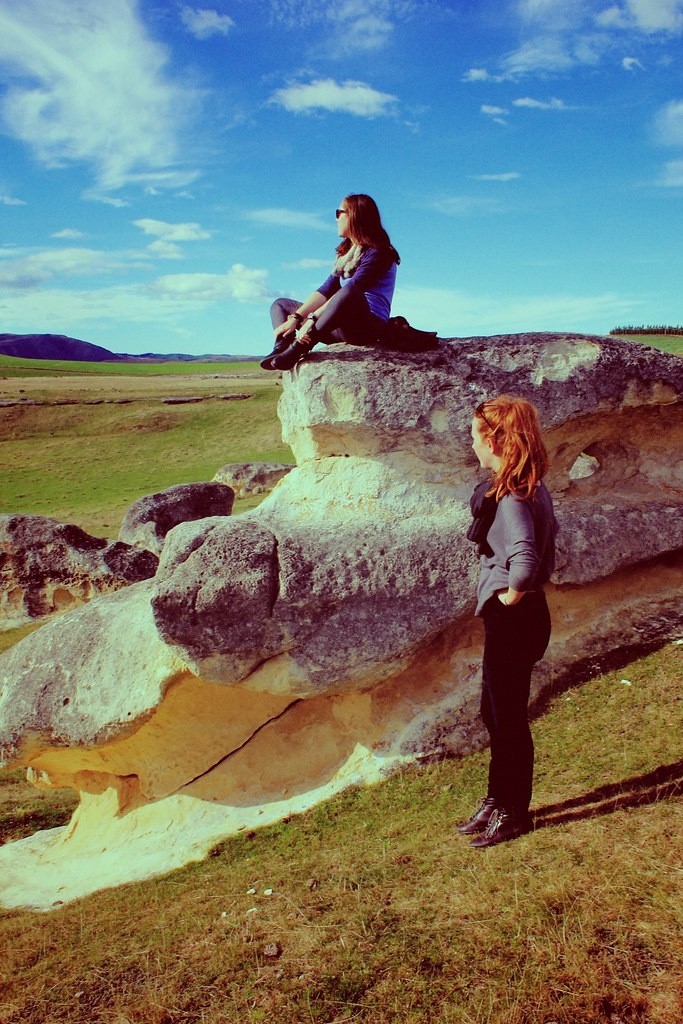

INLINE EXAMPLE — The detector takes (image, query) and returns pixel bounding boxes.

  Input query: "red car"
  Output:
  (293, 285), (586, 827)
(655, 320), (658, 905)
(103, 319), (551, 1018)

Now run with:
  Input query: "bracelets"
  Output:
(287, 312), (303, 324)
(505, 597), (523, 608)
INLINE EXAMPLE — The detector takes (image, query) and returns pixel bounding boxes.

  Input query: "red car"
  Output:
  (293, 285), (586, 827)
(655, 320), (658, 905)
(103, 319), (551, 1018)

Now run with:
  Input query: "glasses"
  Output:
(471, 400), (496, 431)
(335, 208), (348, 218)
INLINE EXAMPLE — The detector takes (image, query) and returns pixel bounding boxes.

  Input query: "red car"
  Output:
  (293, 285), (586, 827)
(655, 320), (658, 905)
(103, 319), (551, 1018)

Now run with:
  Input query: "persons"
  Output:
(259, 193), (401, 370)
(453, 394), (560, 848)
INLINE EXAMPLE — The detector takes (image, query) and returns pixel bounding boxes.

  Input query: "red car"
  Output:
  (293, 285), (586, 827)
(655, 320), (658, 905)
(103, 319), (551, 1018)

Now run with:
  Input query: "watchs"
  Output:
(307, 312), (317, 322)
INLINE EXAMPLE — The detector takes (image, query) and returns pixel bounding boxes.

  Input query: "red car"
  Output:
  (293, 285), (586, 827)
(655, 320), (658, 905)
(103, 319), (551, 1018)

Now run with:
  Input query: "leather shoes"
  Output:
(455, 797), (502, 834)
(270, 338), (311, 371)
(470, 807), (535, 847)
(259, 330), (298, 370)
